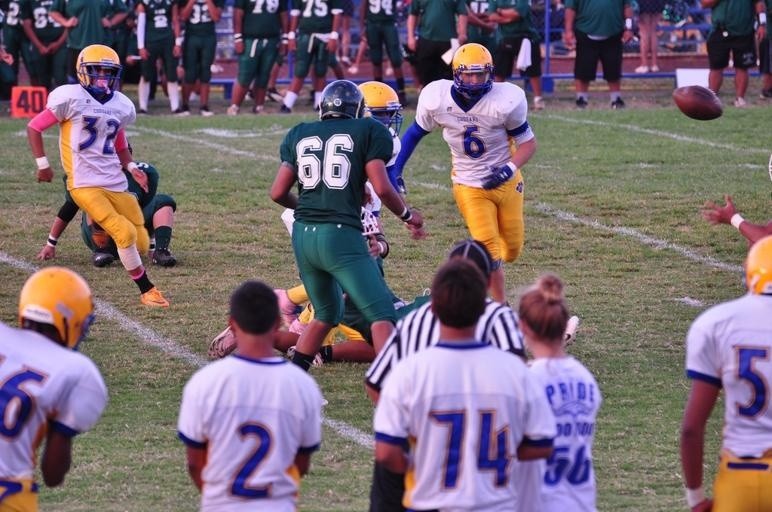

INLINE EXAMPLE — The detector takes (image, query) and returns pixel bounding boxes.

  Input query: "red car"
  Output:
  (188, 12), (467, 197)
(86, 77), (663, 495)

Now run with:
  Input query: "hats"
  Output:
(449, 240), (492, 276)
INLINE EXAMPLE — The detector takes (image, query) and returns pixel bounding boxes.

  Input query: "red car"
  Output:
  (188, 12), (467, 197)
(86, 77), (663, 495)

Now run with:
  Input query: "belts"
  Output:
(725, 462), (767, 470)
(1, 477), (37, 507)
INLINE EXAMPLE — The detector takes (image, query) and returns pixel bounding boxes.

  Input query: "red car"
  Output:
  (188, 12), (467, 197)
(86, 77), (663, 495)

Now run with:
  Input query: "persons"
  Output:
(174, 278), (323, 512)
(367, 256), (557, 511)
(209, 265), (376, 363)
(365, 239), (579, 406)
(0, 265), (109, 512)
(35, 143), (177, 268)
(387, 43), (538, 308)
(512, 274), (601, 511)
(27, 44), (169, 308)
(1, 1), (771, 115)
(702, 191), (772, 244)
(681, 236), (772, 511)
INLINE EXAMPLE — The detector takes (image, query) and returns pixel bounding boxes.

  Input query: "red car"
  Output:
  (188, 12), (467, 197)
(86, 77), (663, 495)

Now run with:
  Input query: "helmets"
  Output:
(451, 43), (496, 100)
(17, 265), (96, 352)
(318, 81), (365, 120)
(76, 45), (124, 98)
(358, 81), (404, 140)
(745, 234), (772, 296)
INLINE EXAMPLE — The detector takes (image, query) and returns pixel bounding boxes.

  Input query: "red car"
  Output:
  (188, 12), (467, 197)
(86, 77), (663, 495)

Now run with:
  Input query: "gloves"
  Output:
(480, 164), (513, 190)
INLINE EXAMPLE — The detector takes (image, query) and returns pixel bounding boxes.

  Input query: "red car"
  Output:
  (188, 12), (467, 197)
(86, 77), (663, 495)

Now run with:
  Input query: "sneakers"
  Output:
(226, 107), (239, 115)
(635, 67), (648, 72)
(200, 109), (214, 115)
(274, 288), (304, 327)
(735, 97), (745, 107)
(140, 285), (170, 308)
(612, 96), (624, 110)
(254, 106), (267, 115)
(575, 97), (588, 111)
(152, 248), (176, 266)
(534, 101), (544, 110)
(280, 104), (291, 114)
(286, 346), (324, 366)
(289, 319), (307, 335)
(93, 250), (115, 267)
(652, 66), (659, 72)
(264, 88), (281, 102)
(173, 106), (189, 116)
(207, 326), (238, 360)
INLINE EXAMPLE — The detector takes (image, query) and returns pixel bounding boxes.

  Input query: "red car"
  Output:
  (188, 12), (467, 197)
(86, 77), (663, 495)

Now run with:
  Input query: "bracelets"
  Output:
(399, 207), (414, 222)
(35, 155), (51, 170)
(730, 212), (744, 231)
(686, 485), (707, 508)
(46, 234), (57, 248)
(127, 161), (140, 174)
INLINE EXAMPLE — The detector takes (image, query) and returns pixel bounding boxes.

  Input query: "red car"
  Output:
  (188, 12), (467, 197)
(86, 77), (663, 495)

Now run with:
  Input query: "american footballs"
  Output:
(674, 85), (722, 120)
